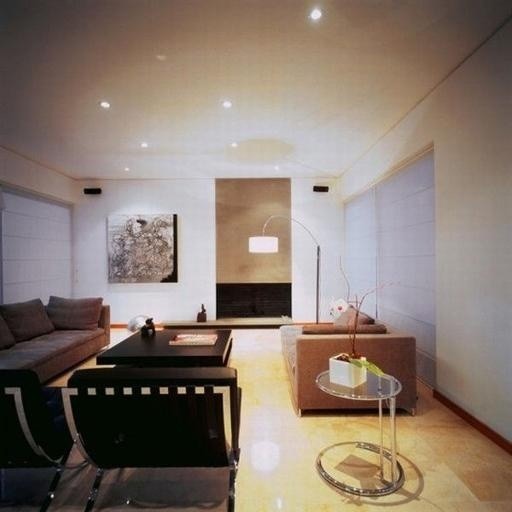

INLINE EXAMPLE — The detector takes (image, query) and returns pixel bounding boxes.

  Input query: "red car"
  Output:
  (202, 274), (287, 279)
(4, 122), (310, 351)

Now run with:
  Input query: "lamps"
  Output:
(247, 215), (321, 324)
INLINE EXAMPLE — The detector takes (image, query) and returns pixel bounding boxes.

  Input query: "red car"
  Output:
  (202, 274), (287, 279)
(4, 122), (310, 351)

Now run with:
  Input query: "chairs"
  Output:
(0, 367), (91, 511)
(67, 366), (243, 512)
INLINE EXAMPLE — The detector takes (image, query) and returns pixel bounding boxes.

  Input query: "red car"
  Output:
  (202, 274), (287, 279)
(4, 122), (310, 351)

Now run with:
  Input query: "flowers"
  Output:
(326, 255), (407, 380)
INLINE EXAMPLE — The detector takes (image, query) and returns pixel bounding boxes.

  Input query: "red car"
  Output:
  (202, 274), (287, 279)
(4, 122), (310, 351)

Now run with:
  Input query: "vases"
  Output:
(329, 352), (368, 389)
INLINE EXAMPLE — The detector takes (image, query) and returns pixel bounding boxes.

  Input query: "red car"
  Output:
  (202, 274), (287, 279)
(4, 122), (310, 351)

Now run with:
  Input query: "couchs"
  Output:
(280, 306), (418, 417)
(1, 296), (113, 385)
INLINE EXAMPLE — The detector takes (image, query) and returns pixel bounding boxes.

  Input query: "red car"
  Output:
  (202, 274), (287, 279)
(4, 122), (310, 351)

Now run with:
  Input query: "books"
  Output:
(169, 333), (219, 346)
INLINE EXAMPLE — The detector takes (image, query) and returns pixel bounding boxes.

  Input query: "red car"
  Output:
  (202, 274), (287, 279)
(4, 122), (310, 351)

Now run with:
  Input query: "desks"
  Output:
(316, 370), (406, 497)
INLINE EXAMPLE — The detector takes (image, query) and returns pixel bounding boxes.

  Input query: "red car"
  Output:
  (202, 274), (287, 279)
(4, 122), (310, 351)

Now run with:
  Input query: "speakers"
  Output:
(313, 185), (329, 192)
(84, 187), (102, 195)
